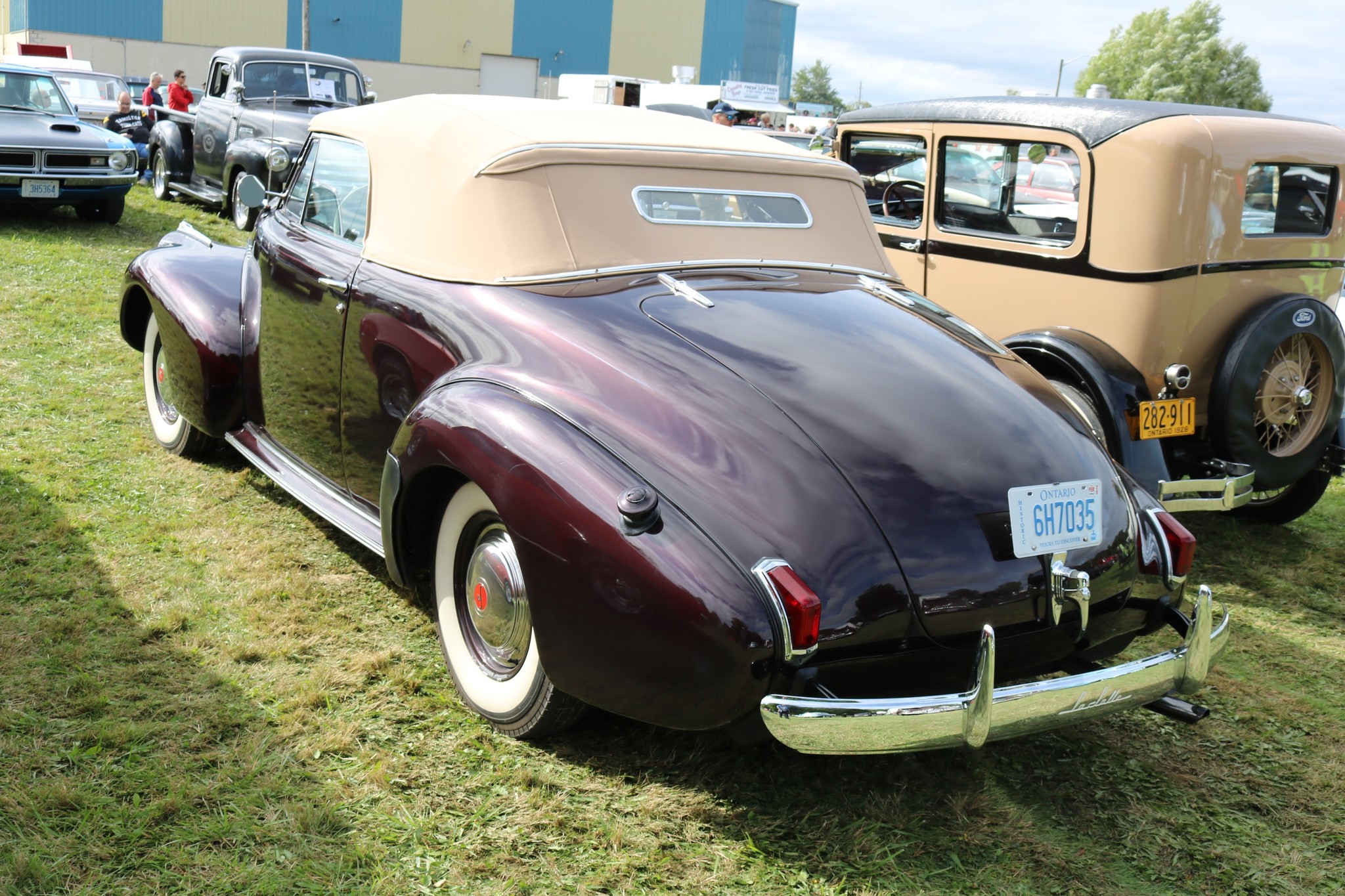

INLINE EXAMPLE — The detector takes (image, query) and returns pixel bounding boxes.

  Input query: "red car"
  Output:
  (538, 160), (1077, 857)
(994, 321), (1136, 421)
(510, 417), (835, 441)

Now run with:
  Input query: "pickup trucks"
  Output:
(148, 46), (377, 230)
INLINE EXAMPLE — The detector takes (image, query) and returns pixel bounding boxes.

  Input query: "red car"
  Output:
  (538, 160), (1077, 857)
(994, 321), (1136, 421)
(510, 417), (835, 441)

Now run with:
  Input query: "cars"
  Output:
(119, 95), (1228, 758)
(0, 63), (139, 226)
(0, 54), (206, 129)
(639, 104), (1280, 221)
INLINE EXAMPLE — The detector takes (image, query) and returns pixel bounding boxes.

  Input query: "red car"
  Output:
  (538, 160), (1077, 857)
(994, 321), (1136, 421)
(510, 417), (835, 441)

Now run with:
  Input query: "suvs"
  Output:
(805, 104), (1345, 527)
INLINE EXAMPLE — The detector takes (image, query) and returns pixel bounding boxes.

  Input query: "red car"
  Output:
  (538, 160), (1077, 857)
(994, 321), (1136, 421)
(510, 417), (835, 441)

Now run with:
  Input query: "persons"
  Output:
(1250, 165), (1273, 195)
(711, 102), (835, 136)
(33, 69), (207, 186)
(1047, 147), (1057, 157)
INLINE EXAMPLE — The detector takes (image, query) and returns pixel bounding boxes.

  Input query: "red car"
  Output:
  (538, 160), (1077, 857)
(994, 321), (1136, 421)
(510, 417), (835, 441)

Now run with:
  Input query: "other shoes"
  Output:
(136, 178), (148, 187)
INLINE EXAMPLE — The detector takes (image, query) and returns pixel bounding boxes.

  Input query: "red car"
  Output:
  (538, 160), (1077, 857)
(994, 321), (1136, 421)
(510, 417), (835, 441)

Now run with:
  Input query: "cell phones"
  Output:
(127, 129), (134, 135)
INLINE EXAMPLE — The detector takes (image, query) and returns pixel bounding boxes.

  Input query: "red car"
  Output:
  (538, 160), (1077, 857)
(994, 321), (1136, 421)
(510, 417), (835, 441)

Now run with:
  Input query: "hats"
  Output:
(747, 118), (757, 123)
(777, 124), (785, 127)
(712, 102), (739, 115)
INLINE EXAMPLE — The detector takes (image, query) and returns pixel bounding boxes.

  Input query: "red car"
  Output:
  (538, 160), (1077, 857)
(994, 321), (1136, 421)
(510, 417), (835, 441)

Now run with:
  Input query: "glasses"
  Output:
(721, 114), (737, 121)
(178, 76), (186, 79)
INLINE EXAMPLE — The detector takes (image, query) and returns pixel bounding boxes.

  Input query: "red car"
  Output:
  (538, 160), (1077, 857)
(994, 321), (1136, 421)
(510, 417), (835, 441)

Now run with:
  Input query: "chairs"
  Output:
(0, 87), (25, 106)
